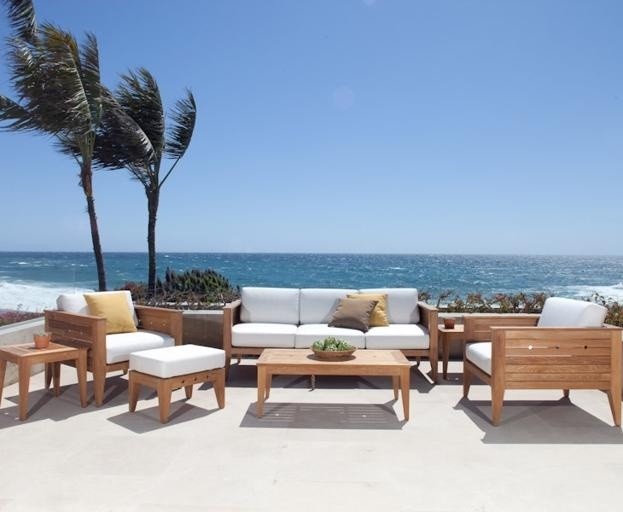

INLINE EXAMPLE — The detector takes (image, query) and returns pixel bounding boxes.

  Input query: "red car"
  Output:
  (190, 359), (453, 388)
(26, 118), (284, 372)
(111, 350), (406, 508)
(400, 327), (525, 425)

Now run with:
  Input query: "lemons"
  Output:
(313, 337), (353, 351)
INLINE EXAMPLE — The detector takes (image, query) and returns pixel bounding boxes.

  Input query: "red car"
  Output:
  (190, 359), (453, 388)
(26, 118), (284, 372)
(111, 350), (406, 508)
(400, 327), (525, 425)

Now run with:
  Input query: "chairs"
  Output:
(43, 288), (184, 406)
(464, 294), (621, 427)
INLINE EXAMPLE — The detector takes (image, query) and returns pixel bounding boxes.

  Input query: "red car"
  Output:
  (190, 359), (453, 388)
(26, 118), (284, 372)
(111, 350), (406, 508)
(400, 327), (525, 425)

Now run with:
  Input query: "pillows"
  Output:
(328, 293), (388, 332)
(84, 291), (138, 335)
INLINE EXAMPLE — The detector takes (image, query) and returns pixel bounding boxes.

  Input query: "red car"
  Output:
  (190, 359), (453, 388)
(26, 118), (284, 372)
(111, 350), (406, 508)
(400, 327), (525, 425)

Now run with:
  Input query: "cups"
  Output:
(33, 333), (52, 348)
(443, 317), (456, 329)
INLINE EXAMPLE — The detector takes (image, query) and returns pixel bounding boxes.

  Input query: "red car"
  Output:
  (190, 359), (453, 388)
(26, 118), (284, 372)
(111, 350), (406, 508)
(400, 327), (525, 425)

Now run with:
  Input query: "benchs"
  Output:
(221, 287), (440, 383)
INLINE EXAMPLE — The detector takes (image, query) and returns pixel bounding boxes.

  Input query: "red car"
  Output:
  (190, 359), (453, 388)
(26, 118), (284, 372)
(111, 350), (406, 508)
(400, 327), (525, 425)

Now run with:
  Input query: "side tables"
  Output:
(436, 322), (466, 380)
(1, 342), (90, 422)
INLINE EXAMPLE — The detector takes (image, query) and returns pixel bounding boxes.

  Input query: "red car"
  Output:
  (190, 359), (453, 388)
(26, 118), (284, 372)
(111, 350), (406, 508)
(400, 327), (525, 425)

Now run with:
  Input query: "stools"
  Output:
(126, 344), (226, 423)
